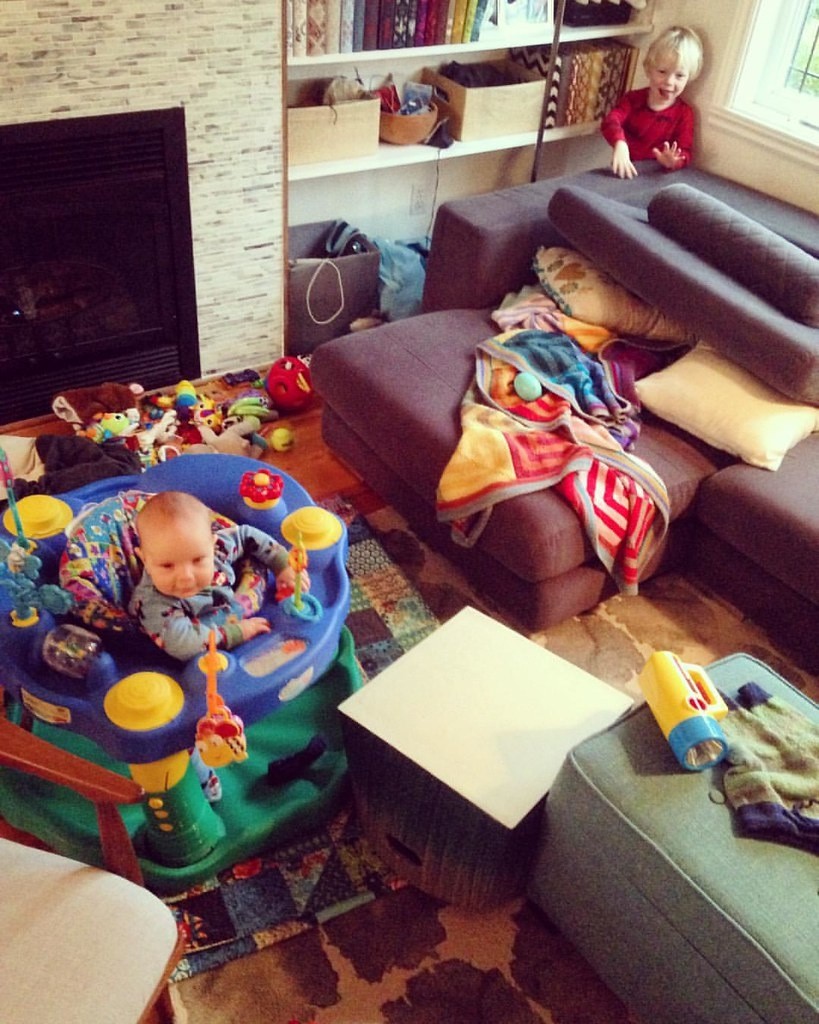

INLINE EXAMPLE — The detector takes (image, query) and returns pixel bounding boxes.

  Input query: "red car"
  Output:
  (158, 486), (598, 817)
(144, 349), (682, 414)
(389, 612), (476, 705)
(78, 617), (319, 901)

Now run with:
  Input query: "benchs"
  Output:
(523, 649), (819, 1024)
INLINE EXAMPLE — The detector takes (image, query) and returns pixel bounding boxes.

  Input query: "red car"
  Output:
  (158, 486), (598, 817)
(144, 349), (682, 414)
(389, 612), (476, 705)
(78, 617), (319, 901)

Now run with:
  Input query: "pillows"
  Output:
(531, 246), (694, 345)
(635, 336), (819, 472)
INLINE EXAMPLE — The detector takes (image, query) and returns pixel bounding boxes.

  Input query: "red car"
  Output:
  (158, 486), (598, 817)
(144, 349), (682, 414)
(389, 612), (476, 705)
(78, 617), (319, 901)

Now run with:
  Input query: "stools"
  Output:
(0, 835), (180, 1024)
(336, 606), (636, 917)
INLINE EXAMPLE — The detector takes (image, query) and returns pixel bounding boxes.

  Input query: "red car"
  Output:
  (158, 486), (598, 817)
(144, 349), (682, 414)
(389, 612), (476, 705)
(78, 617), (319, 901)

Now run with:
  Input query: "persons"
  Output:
(128, 491), (311, 803)
(601, 25), (704, 179)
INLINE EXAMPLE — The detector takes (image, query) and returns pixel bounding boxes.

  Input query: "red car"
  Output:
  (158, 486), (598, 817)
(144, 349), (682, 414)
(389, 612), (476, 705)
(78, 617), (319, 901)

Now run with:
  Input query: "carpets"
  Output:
(175, 505), (819, 1024)
(167, 489), (442, 989)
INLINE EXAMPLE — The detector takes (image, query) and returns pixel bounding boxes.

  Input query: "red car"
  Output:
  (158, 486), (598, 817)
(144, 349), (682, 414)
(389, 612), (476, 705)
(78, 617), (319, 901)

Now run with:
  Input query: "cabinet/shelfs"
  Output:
(287, 24), (653, 186)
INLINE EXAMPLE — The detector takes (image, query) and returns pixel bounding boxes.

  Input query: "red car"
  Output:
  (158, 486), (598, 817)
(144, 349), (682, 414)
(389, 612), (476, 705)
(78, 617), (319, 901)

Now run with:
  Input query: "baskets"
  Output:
(379, 101), (438, 145)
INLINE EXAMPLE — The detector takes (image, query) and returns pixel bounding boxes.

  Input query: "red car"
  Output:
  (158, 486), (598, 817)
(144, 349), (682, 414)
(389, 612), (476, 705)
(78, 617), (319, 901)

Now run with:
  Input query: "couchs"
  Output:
(303, 159), (819, 677)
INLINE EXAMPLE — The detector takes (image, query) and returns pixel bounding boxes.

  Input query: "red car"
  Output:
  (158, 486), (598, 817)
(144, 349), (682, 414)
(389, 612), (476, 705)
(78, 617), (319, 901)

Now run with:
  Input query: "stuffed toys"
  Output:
(75, 355), (313, 465)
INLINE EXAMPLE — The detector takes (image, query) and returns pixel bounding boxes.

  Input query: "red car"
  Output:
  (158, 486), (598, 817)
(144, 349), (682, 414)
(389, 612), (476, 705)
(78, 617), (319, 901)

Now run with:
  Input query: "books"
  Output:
(285, 0), (488, 57)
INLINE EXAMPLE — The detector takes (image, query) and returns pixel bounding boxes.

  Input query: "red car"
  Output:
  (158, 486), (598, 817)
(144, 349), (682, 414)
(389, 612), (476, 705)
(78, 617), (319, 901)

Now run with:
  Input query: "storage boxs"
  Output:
(509, 40), (640, 130)
(289, 218), (382, 358)
(285, 75), (381, 168)
(423, 59), (547, 142)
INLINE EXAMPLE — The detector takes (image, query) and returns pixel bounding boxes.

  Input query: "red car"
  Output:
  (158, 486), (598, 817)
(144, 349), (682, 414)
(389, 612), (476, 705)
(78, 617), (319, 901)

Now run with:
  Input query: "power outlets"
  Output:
(410, 183), (429, 215)
